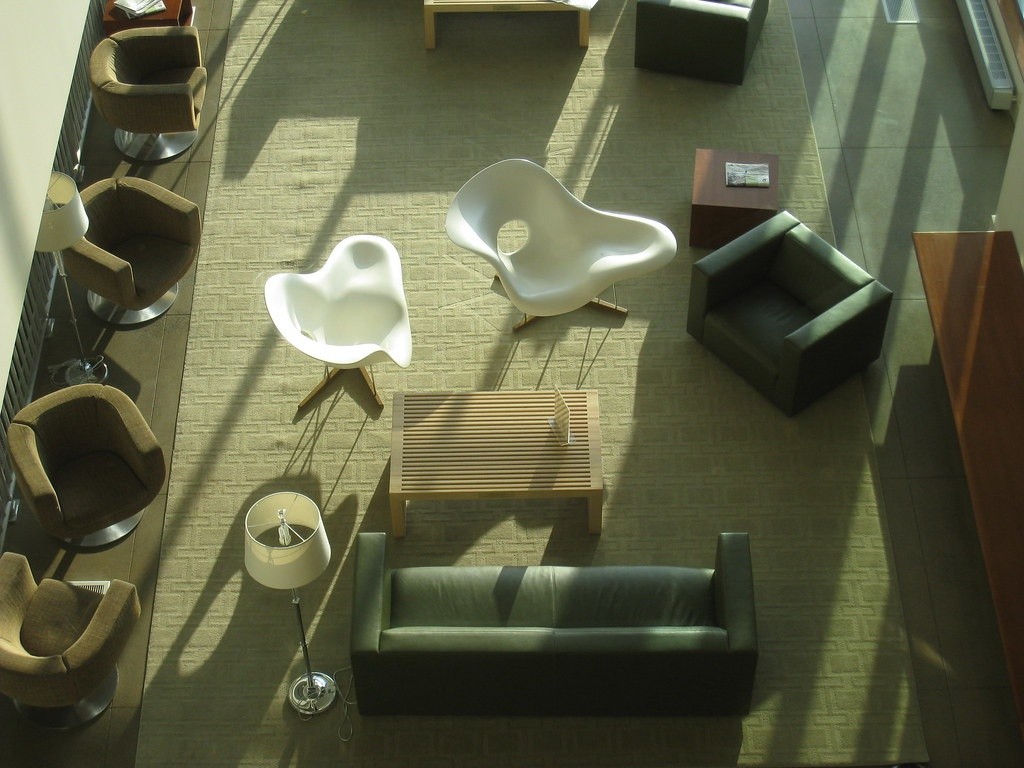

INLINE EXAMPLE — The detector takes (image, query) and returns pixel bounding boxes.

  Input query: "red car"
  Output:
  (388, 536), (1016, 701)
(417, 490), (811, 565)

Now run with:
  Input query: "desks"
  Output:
(391, 388), (605, 539)
(688, 147), (779, 248)
(103, 0), (195, 34)
(911, 231), (1023, 719)
(423, 1), (591, 50)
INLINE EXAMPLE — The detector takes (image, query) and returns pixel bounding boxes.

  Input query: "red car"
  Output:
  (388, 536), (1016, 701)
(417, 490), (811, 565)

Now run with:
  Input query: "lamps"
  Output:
(243, 492), (340, 717)
(39, 171), (111, 388)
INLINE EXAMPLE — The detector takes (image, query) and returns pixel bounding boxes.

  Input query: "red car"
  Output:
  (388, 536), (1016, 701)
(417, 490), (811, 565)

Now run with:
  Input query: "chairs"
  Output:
(445, 157), (678, 332)
(632, 0), (771, 85)
(70, 178), (201, 328)
(0, 552), (142, 731)
(12, 382), (166, 549)
(92, 26), (207, 162)
(264, 236), (412, 410)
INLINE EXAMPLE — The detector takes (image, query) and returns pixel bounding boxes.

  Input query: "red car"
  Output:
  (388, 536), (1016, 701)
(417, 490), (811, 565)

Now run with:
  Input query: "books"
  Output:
(114, 0), (166, 19)
(726, 162), (769, 187)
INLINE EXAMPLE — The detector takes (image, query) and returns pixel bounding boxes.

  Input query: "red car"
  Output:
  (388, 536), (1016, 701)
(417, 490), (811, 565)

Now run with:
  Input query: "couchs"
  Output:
(356, 530), (757, 719)
(688, 208), (894, 413)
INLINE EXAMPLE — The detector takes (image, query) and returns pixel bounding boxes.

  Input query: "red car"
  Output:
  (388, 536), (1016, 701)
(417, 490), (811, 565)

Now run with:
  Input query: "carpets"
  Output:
(136, 1), (931, 766)
